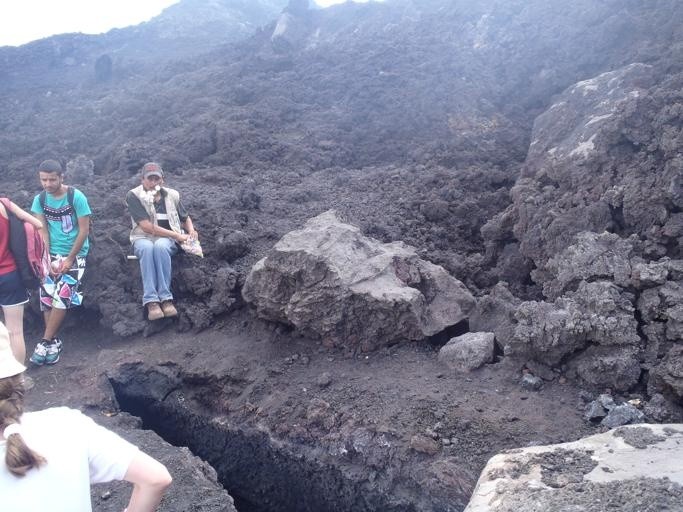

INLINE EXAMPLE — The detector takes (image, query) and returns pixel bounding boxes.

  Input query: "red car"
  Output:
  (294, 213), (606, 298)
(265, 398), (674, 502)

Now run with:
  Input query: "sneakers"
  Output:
(145, 303), (164, 320)
(23, 377), (35, 390)
(161, 300), (177, 317)
(45, 337), (63, 364)
(29, 340), (48, 366)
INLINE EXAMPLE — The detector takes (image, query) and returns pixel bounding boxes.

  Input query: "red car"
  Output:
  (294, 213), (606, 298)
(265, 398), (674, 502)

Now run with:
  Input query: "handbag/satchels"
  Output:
(1, 198), (43, 290)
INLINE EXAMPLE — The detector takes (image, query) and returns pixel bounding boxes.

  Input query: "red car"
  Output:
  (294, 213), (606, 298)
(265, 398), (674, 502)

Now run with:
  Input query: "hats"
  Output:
(0, 321), (27, 379)
(142, 162), (164, 179)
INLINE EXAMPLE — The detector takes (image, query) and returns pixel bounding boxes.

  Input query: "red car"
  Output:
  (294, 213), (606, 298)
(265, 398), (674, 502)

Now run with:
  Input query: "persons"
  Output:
(30, 160), (91, 366)
(0, 195), (42, 388)
(126, 162), (199, 319)
(0, 323), (173, 512)
